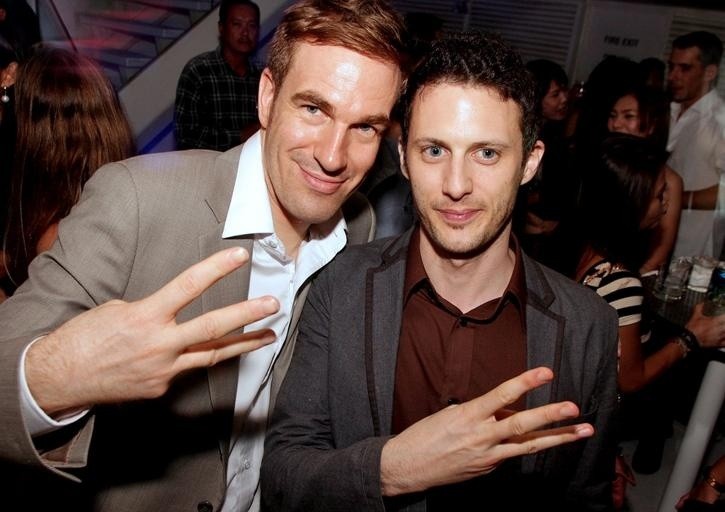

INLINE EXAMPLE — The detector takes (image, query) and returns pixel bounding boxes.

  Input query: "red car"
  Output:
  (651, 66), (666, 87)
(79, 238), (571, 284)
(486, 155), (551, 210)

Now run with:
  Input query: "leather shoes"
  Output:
(631, 438), (665, 475)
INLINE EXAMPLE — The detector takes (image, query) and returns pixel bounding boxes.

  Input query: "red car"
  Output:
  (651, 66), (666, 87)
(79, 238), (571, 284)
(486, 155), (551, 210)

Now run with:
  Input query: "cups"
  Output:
(652, 257), (693, 304)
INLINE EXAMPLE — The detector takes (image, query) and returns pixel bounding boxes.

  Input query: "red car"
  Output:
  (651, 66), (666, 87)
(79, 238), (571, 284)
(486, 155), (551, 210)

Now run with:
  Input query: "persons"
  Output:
(0, 0), (44, 133)
(0, 46), (140, 303)
(258, 29), (621, 511)
(1, 2), (402, 512)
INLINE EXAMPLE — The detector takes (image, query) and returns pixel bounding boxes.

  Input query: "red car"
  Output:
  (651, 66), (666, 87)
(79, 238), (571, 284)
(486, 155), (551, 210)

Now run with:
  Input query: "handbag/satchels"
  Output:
(612, 454), (636, 508)
